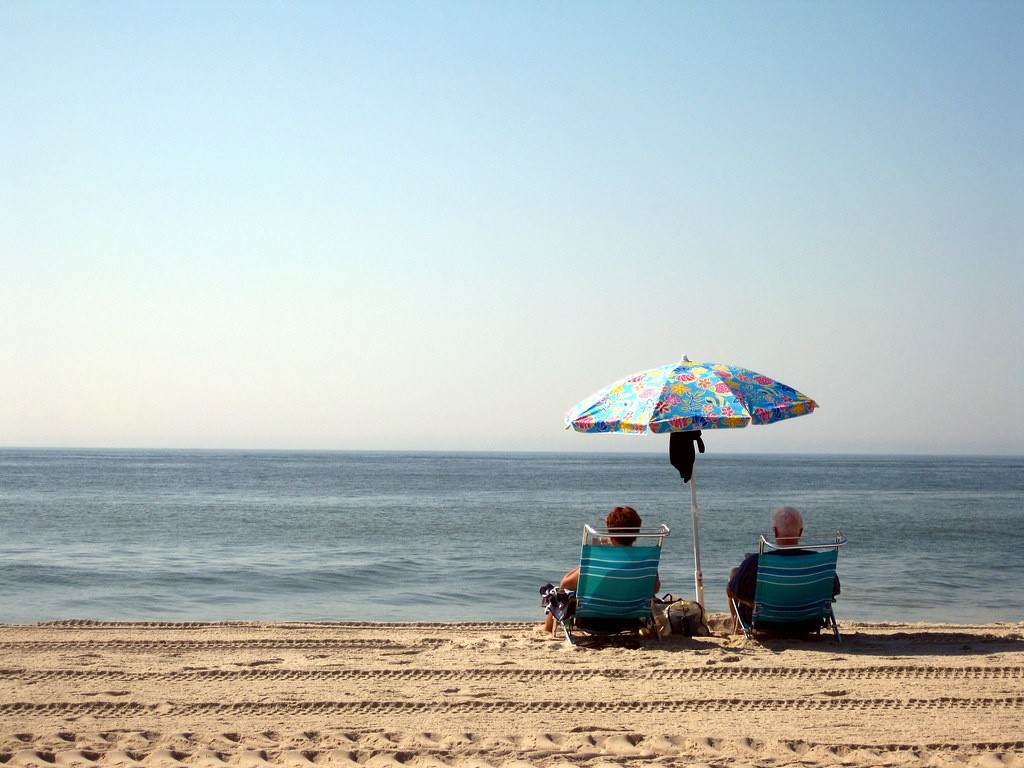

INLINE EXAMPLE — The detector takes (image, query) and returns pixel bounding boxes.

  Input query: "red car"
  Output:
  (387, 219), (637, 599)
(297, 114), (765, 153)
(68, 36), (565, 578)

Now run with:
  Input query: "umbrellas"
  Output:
(565, 354), (820, 607)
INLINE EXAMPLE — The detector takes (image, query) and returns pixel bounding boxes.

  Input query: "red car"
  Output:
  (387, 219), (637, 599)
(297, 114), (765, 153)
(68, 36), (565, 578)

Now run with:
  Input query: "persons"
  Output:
(728, 507), (841, 641)
(544, 506), (660, 633)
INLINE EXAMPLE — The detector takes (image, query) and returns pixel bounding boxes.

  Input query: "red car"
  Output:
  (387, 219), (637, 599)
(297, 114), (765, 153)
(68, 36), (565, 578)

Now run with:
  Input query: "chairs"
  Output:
(727, 529), (848, 647)
(550, 523), (668, 644)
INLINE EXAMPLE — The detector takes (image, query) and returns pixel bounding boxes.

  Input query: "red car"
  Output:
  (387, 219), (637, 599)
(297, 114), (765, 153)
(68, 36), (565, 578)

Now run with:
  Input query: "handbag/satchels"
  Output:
(642, 593), (704, 638)
